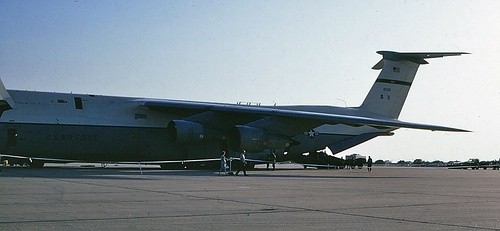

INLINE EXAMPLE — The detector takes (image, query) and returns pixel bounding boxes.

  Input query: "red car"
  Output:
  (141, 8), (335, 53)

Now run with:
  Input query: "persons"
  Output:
(366, 156), (372, 172)
(219, 151), (229, 175)
(235, 150), (247, 175)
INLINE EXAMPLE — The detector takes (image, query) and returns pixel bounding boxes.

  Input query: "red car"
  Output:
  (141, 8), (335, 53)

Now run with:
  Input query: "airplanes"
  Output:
(0, 50), (474, 169)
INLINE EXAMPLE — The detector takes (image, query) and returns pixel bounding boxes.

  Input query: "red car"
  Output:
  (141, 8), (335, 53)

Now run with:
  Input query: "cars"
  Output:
(412, 158), (500, 170)
